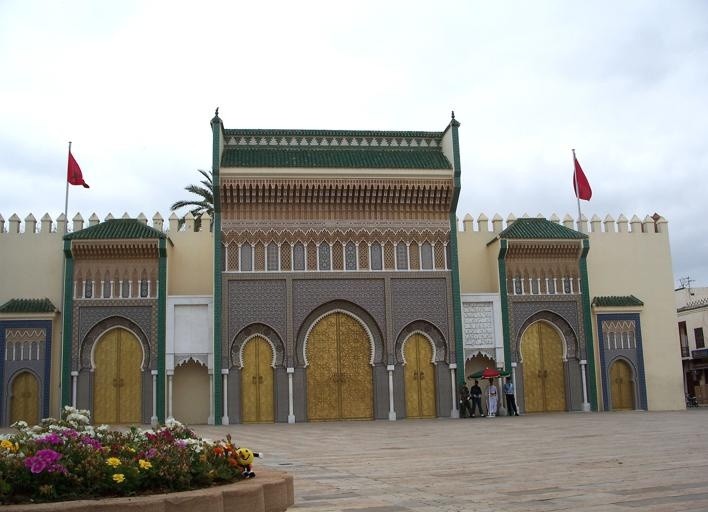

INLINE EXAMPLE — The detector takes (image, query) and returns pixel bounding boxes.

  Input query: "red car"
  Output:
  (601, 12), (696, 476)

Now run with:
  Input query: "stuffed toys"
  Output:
(235, 448), (264, 477)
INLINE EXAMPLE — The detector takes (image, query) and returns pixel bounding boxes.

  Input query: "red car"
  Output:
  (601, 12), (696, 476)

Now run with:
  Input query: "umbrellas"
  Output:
(466, 366), (510, 402)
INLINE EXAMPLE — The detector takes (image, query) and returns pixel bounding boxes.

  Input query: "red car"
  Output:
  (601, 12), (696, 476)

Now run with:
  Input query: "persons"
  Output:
(459, 382), (474, 418)
(503, 376), (520, 416)
(469, 380), (486, 417)
(485, 378), (499, 416)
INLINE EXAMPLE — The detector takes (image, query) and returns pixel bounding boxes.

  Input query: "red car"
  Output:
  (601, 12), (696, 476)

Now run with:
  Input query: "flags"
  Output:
(67, 150), (91, 189)
(571, 156), (593, 202)
